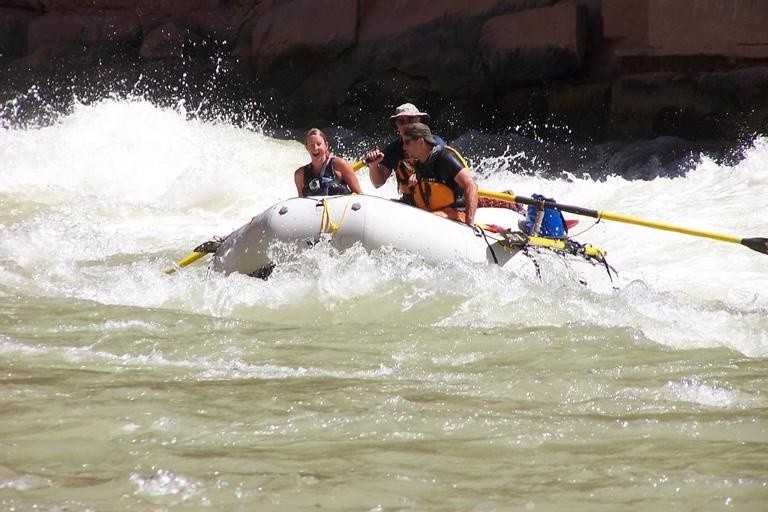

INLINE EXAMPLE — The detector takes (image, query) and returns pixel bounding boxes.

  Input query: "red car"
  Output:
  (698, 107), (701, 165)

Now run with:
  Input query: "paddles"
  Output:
(479, 188), (768, 266)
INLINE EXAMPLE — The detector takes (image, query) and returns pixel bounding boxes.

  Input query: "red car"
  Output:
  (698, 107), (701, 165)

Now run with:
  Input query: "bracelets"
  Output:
(465, 222), (475, 228)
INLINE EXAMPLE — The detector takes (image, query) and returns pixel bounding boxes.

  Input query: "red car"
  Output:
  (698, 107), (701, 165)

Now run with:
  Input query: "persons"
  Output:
(367, 101), (447, 207)
(293, 127), (365, 196)
(402, 122), (479, 229)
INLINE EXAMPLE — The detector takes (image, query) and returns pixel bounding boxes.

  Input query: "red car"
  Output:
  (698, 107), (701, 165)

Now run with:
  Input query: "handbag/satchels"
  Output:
(526, 194), (564, 239)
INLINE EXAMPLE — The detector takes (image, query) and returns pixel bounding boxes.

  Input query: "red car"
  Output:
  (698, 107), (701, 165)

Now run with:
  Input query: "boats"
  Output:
(205, 191), (618, 290)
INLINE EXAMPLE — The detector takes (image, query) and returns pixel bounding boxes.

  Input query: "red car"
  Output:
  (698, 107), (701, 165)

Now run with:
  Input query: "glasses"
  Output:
(404, 139), (414, 145)
(398, 117), (416, 124)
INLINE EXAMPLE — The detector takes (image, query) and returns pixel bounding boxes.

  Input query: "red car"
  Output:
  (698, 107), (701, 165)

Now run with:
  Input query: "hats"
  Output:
(404, 123), (436, 143)
(387, 103), (430, 127)
